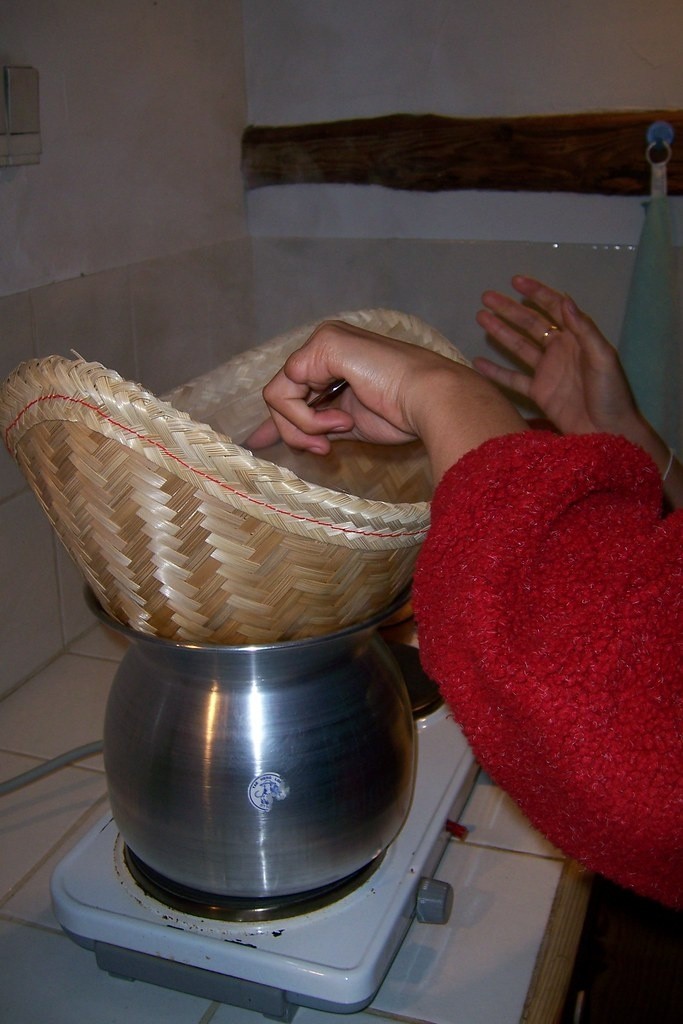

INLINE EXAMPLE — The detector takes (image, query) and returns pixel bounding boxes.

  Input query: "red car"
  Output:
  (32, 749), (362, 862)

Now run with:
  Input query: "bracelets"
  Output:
(661, 450), (675, 482)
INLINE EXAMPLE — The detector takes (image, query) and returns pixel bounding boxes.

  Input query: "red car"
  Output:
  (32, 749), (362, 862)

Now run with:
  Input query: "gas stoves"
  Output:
(51, 621), (481, 1024)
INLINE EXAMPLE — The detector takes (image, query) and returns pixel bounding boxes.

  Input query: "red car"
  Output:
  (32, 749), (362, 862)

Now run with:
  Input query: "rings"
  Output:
(540, 326), (556, 345)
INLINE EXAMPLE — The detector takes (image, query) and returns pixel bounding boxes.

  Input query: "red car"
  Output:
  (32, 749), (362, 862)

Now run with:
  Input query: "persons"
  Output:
(244, 275), (683, 911)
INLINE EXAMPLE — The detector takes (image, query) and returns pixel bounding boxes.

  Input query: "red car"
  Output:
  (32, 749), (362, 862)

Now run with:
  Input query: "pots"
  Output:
(85, 591), (418, 895)
